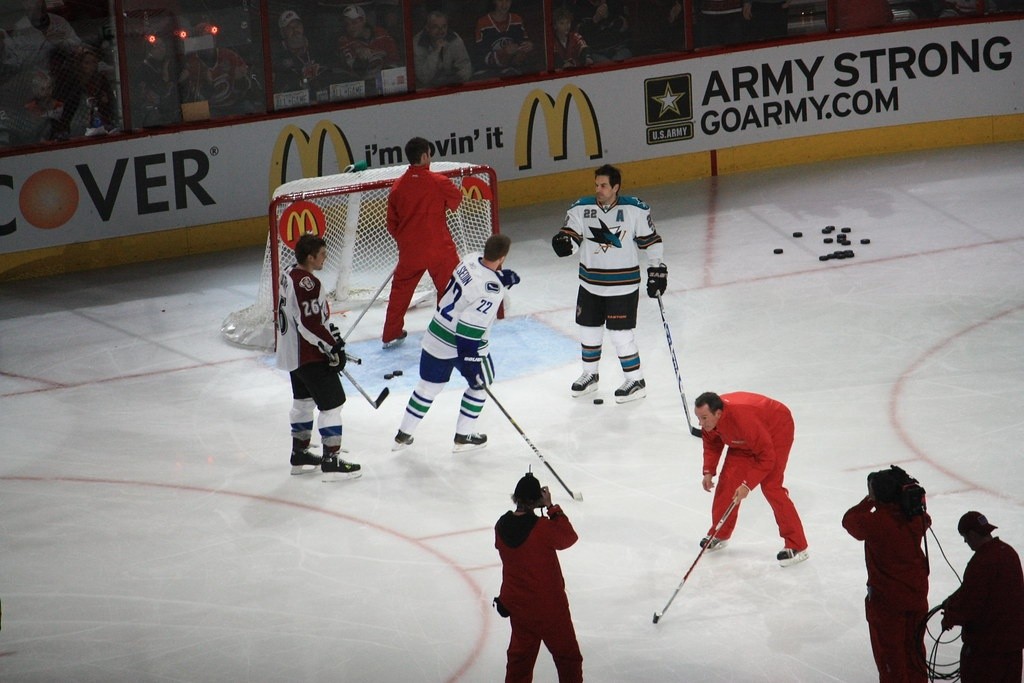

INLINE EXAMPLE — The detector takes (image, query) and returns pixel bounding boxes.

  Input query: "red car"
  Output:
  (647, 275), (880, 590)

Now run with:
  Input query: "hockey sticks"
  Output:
(653, 494), (739, 623)
(655, 289), (702, 437)
(483, 385), (583, 501)
(340, 367), (390, 410)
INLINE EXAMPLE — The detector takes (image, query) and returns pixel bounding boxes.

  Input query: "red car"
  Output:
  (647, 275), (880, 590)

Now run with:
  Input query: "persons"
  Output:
(938, 0), (996, 22)
(695, 0), (792, 46)
(551, 164), (668, 405)
(380, 137), (463, 349)
(414, 9), (473, 89)
(842, 466), (933, 683)
(276, 234), (362, 482)
(695, 392), (808, 567)
(941, 511), (1024, 683)
(122, 0), (249, 129)
(390, 235), (520, 453)
(494, 467), (584, 683)
(548, 0), (682, 71)
(271, 5), (399, 99)
(0, 0), (116, 143)
(474, 0), (531, 77)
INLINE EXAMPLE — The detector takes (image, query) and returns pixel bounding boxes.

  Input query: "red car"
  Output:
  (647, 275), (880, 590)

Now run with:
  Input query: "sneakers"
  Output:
(392, 431), (414, 451)
(321, 445), (362, 482)
(571, 371), (599, 398)
(453, 429), (488, 453)
(778, 544), (808, 567)
(290, 437), (325, 474)
(382, 329), (407, 348)
(614, 378), (646, 403)
(700, 536), (727, 554)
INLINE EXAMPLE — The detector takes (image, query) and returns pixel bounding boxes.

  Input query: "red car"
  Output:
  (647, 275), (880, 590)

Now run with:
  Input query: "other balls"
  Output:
(826, 226), (835, 231)
(824, 238), (833, 243)
(837, 234), (847, 242)
(793, 232), (802, 237)
(843, 240), (851, 245)
(594, 399), (603, 404)
(822, 228), (831, 233)
(392, 371), (402, 376)
(384, 374), (394, 379)
(841, 227), (851, 233)
(774, 249), (783, 253)
(819, 249), (855, 261)
(861, 239), (870, 244)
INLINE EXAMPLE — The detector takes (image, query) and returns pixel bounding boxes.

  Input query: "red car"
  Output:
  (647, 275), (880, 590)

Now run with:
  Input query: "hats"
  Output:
(278, 10), (301, 29)
(514, 475), (541, 500)
(957, 512), (997, 536)
(342, 7), (366, 22)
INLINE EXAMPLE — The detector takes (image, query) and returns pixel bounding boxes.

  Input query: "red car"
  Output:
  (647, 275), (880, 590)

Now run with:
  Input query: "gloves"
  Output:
(551, 230), (574, 258)
(325, 345), (346, 373)
(329, 323), (345, 349)
(647, 266), (670, 301)
(495, 268), (520, 290)
